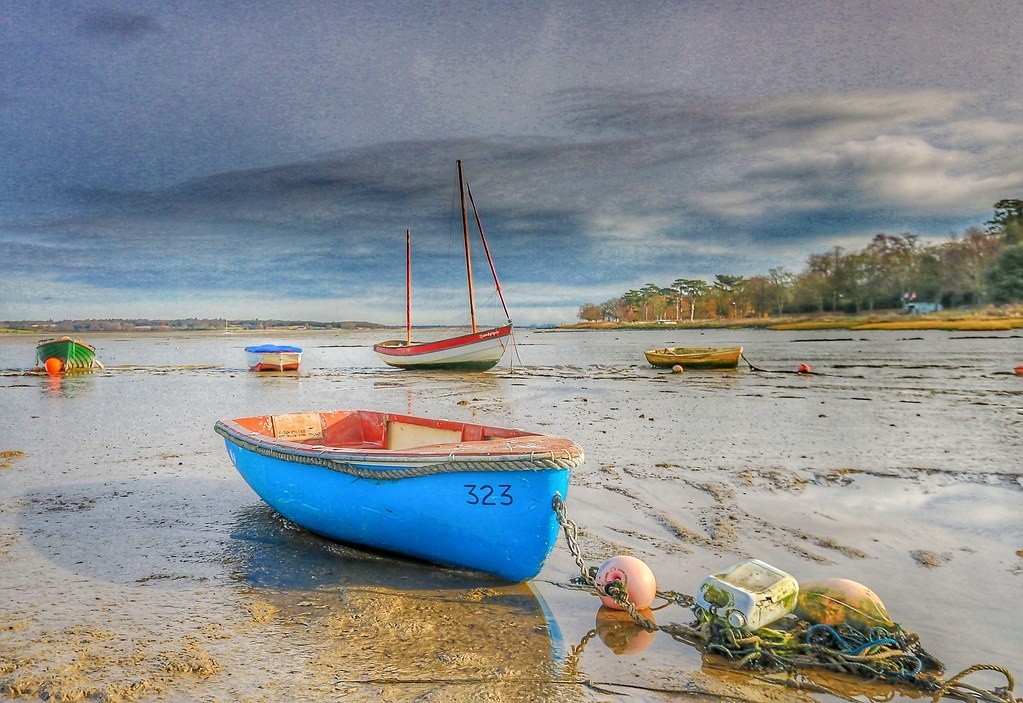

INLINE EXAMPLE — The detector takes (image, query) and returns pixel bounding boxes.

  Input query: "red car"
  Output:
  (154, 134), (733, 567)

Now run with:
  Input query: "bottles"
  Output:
(696, 558), (798, 632)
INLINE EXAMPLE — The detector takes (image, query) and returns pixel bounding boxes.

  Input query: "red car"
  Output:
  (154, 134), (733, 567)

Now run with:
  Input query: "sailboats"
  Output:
(372, 155), (514, 374)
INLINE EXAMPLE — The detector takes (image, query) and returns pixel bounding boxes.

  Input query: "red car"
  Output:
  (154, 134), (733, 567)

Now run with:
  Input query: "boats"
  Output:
(245, 343), (303, 374)
(898, 301), (945, 315)
(644, 345), (746, 369)
(210, 405), (588, 584)
(35, 336), (98, 377)
